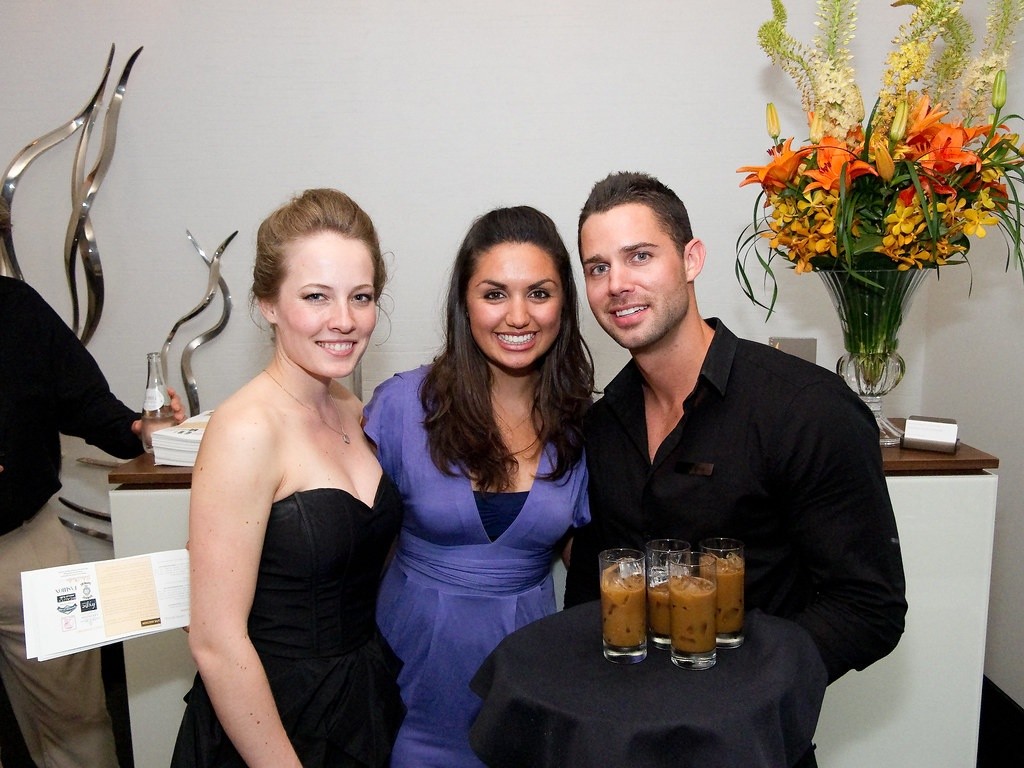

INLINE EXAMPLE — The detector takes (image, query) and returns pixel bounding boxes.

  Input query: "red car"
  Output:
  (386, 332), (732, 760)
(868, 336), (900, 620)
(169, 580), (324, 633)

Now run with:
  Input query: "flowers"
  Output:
(732, 0), (1023, 397)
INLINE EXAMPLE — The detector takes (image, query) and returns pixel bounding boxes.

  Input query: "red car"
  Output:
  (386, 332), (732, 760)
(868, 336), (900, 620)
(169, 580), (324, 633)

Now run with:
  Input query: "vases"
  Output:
(812, 270), (906, 450)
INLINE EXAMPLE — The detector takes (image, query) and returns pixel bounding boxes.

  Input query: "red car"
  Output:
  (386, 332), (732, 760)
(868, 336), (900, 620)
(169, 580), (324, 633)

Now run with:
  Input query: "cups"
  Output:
(599, 550), (647, 664)
(669, 551), (717, 672)
(645, 539), (691, 651)
(698, 539), (747, 650)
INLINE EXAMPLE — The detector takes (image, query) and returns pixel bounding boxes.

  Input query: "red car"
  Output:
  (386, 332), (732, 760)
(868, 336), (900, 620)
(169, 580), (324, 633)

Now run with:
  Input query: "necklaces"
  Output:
(262, 369), (351, 445)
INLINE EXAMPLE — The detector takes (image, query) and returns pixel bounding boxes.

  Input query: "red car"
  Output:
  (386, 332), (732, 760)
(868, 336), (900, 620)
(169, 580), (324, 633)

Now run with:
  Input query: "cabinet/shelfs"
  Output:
(106, 416), (1001, 768)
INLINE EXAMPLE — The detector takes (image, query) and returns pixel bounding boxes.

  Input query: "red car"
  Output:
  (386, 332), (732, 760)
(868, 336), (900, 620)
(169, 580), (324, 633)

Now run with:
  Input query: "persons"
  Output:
(560, 170), (910, 688)
(354, 201), (595, 768)
(0, 274), (189, 768)
(169, 188), (409, 768)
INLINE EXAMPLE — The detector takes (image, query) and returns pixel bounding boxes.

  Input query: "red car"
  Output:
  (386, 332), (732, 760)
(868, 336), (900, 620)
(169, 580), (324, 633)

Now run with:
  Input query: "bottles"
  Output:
(142, 352), (175, 455)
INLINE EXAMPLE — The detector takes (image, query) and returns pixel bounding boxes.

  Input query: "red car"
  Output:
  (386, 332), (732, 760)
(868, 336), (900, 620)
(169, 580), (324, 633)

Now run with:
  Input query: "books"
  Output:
(149, 408), (216, 467)
